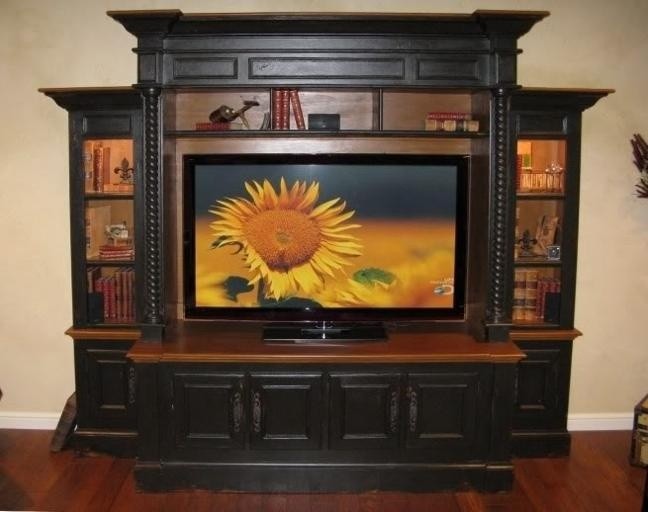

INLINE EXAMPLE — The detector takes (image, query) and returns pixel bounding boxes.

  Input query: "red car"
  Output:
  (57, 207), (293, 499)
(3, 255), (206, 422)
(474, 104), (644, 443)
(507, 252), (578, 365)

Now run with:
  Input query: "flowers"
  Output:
(205, 177), (365, 312)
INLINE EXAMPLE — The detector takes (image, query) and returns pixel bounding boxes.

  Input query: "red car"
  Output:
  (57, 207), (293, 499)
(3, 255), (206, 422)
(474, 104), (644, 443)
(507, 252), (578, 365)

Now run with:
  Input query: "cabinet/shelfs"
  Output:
(64, 328), (142, 458)
(511, 87), (616, 330)
(38, 86), (142, 328)
(512, 328), (581, 459)
(106, 10), (553, 136)
(127, 338), (527, 494)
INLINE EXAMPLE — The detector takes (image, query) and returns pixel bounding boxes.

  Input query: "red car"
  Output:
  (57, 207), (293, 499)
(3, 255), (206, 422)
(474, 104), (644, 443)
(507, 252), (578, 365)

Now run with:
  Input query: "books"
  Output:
(196, 121), (226, 131)
(513, 269), (560, 324)
(273, 89), (305, 131)
(427, 113), (468, 120)
(86, 208), (135, 324)
(426, 119), (479, 132)
(517, 154), (523, 191)
(83, 142), (134, 192)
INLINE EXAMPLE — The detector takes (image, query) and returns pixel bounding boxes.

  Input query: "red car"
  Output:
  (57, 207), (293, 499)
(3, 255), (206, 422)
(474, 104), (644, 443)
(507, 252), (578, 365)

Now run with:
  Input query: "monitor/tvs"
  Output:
(182, 152), (472, 341)
